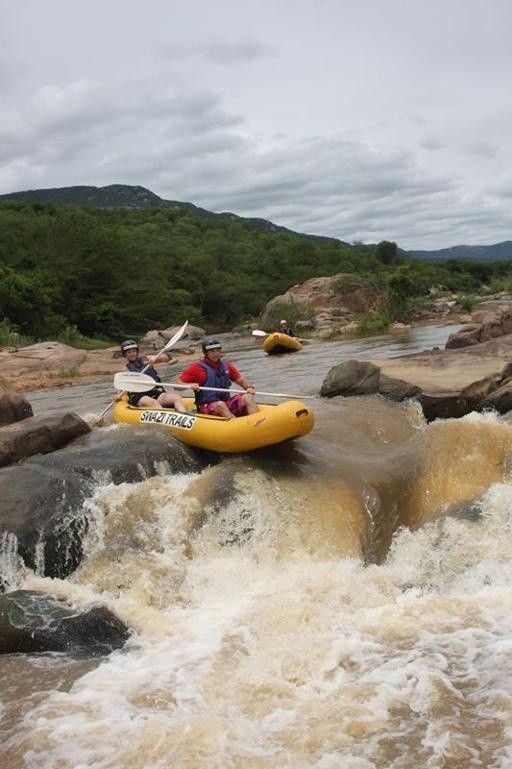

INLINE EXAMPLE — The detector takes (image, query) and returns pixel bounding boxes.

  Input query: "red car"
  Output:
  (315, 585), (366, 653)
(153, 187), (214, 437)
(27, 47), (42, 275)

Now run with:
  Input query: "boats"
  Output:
(113, 393), (315, 453)
(263, 332), (302, 354)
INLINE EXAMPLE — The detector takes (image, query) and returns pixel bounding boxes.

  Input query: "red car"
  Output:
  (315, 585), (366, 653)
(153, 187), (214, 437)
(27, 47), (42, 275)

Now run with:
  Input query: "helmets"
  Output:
(121, 339), (139, 357)
(280, 319), (287, 324)
(201, 339), (222, 355)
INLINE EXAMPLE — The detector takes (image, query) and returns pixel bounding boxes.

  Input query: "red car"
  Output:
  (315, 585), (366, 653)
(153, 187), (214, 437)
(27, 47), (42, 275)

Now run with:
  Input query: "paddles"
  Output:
(113, 371), (311, 402)
(252, 330), (310, 342)
(96, 320), (188, 423)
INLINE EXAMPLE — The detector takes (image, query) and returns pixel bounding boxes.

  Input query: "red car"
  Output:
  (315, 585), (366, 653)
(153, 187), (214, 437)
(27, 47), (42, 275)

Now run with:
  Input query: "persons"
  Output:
(278, 320), (294, 337)
(174, 338), (259, 418)
(113, 340), (188, 413)
